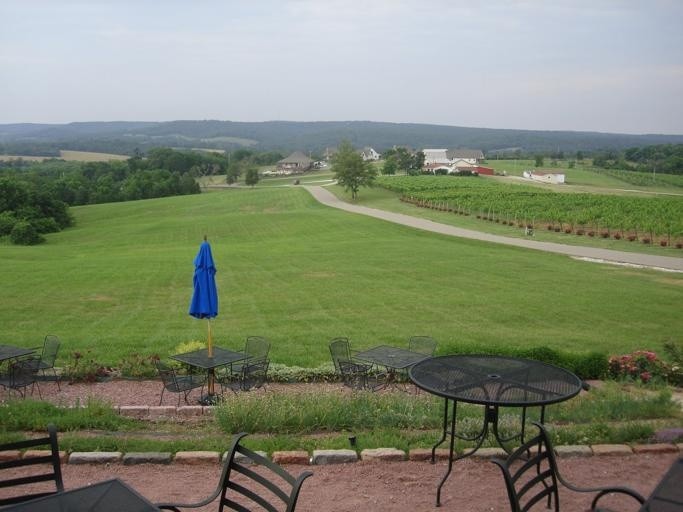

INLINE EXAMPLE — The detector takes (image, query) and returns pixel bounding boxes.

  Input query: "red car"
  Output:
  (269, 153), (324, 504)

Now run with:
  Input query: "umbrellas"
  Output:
(186, 237), (226, 359)
